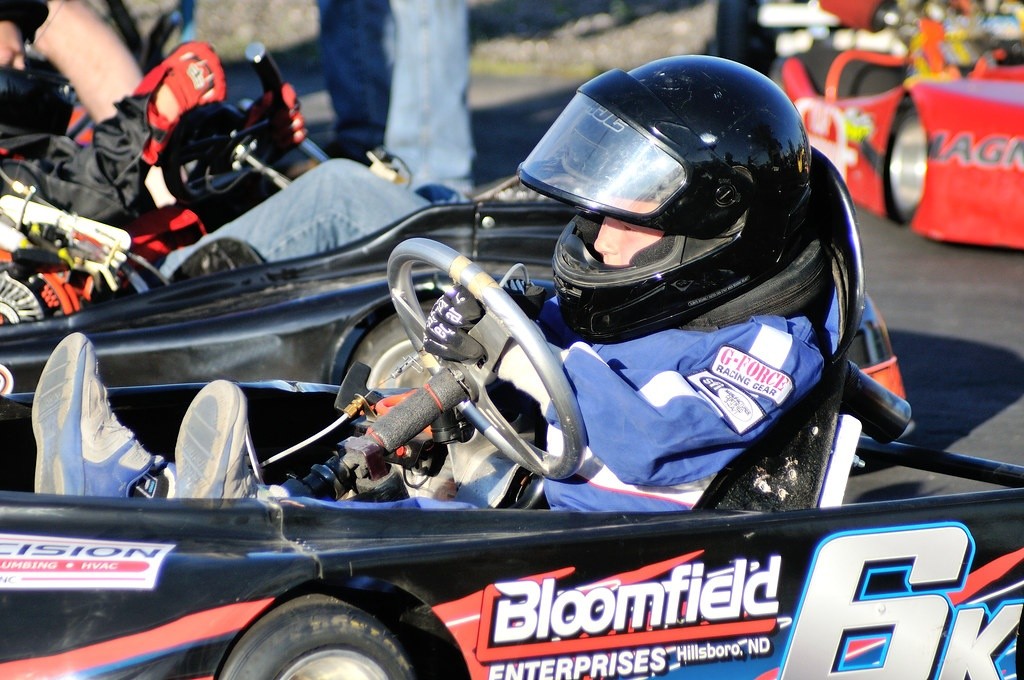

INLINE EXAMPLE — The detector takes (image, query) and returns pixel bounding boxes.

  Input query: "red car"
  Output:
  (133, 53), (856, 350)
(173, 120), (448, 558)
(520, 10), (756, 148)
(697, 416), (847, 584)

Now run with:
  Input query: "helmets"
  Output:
(517, 55), (811, 343)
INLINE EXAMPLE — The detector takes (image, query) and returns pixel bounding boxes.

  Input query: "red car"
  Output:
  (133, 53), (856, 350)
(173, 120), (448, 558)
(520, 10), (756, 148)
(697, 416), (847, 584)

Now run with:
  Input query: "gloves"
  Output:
(241, 84), (308, 166)
(421, 284), (485, 363)
(134, 40), (225, 118)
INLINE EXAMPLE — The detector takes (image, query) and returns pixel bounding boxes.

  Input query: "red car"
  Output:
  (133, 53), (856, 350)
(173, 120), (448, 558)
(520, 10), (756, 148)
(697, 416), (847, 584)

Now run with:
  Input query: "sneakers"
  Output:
(31, 332), (164, 497)
(175, 378), (261, 500)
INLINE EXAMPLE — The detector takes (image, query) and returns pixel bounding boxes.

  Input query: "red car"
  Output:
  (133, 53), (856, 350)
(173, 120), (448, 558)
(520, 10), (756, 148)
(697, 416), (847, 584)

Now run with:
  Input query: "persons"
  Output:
(31, 54), (825, 510)
(315, 0), (476, 204)
(0, 0), (432, 285)
